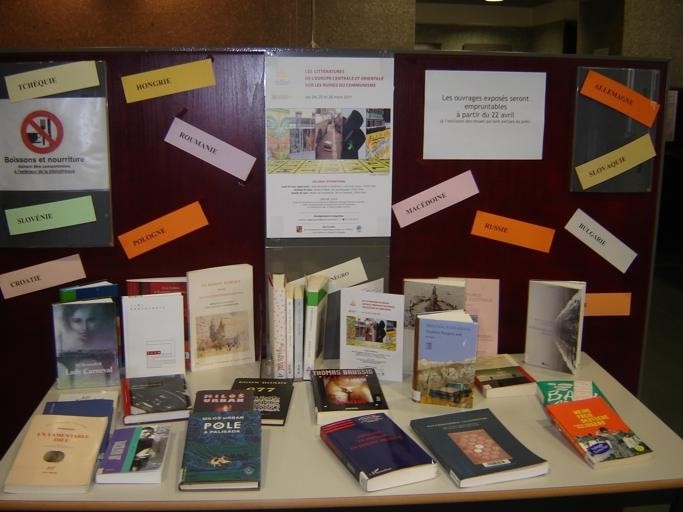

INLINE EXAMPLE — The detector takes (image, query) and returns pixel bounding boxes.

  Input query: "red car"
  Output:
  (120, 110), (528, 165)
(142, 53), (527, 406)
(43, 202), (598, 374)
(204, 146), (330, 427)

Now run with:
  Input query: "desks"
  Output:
(1, 350), (682, 511)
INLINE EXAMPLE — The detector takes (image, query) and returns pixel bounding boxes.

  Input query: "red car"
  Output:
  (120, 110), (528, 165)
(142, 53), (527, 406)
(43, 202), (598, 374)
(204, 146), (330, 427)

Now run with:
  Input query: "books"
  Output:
(1, 257), (654, 494)
